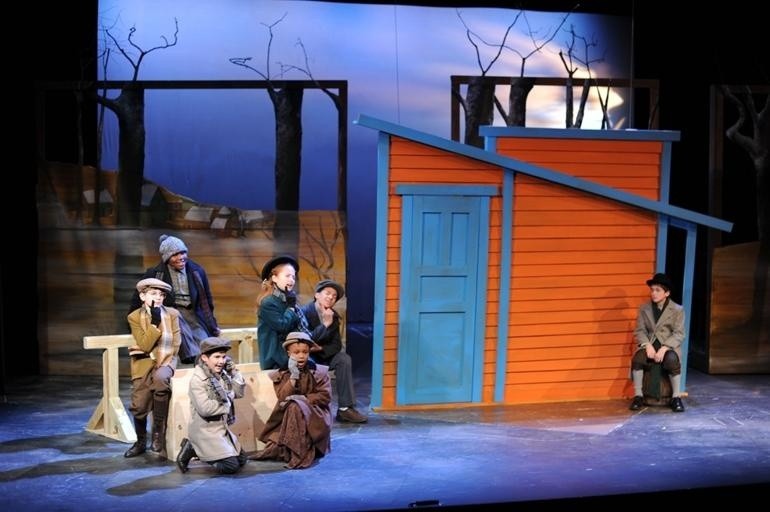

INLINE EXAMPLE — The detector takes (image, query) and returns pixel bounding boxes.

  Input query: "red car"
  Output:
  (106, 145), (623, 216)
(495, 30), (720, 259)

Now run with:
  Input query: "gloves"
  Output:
(149, 301), (161, 325)
(285, 286), (296, 307)
(223, 355), (235, 373)
(288, 356), (300, 379)
(224, 389), (235, 407)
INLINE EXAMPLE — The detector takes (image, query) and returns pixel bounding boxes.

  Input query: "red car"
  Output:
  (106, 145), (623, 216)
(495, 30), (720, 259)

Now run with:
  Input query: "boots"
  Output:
(124, 418), (147, 458)
(151, 400), (169, 452)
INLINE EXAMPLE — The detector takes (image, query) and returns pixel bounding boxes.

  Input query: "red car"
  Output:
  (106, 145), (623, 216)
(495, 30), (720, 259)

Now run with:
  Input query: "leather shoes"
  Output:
(670, 397), (684, 411)
(336, 407), (368, 422)
(630, 396), (642, 410)
(177, 438), (197, 473)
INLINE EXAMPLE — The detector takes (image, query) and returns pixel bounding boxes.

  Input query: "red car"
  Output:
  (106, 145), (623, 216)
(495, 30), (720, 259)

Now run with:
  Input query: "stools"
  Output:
(641, 361), (673, 407)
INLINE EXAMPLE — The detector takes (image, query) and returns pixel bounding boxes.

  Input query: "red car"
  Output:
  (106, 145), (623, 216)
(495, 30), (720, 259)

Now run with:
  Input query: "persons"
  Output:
(248, 331), (332, 470)
(255, 255), (318, 369)
(123, 276), (182, 459)
(177, 336), (248, 473)
(630, 273), (685, 411)
(298, 278), (368, 424)
(128, 234), (221, 363)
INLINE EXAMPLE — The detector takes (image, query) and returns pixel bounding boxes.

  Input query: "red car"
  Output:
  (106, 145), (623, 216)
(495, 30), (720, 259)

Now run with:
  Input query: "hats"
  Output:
(315, 279), (344, 300)
(647, 274), (678, 289)
(158, 234), (189, 263)
(282, 332), (316, 351)
(135, 278), (172, 293)
(199, 337), (231, 355)
(262, 254), (299, 280)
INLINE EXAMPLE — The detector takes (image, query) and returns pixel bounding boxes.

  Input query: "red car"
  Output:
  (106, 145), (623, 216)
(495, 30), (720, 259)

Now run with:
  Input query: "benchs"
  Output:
(152, 362), (279, 463)
